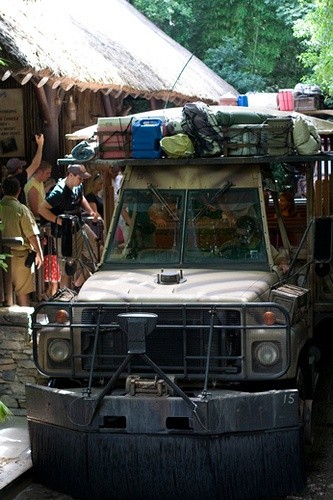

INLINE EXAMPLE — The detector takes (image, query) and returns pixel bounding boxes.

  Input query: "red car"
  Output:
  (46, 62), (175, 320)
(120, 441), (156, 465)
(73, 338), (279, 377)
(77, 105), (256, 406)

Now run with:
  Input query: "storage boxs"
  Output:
(294, 95), (320, 110)
(96, 126), (132, 159)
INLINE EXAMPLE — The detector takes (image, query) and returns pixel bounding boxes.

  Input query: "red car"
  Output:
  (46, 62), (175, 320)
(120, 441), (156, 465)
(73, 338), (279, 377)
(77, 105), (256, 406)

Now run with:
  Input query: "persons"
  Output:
(36, 208), (62, 301)
(0, 176), (44, 307)
(36, 165), (99, 297)
(221, 216), (292, 275)
(113, 166), (167, 249)
(23, 163), (51, 223)
(0, 133), (56, 206)
(73, 174), (105, 289)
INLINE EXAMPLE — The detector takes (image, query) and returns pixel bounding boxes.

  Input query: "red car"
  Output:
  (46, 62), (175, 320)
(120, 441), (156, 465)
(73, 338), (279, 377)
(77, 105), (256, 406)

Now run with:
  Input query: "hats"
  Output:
(7, 158), (27, 169)
(67, 164), (92, 180)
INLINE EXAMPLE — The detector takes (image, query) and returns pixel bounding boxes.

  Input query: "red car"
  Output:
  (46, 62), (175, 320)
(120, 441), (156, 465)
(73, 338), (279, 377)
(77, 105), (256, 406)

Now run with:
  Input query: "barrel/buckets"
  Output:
(131, 118), (162, 159)
(238, 95), (248, 107)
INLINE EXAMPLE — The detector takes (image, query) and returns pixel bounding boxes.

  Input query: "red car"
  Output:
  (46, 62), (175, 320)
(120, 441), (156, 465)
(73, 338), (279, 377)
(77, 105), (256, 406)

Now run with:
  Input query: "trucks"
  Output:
(18, 80), (333, 500)
